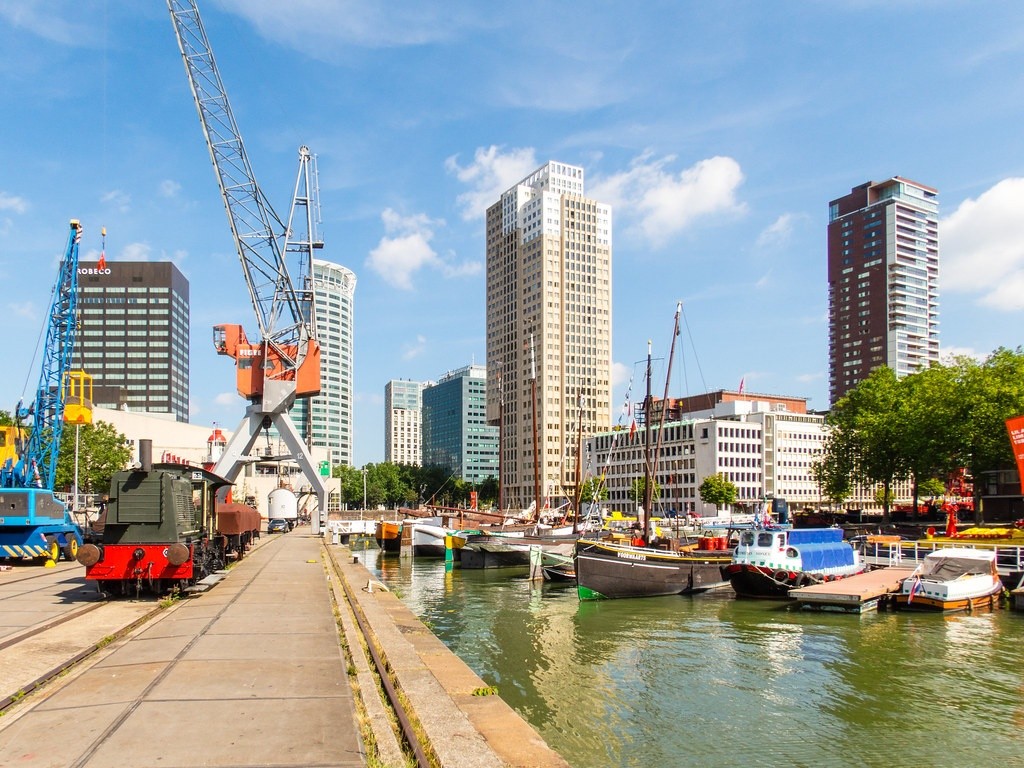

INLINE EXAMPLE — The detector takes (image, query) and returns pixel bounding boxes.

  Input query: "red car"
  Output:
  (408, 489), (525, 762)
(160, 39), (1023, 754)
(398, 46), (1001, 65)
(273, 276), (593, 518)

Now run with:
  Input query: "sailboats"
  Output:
(376, 300), (763, 596)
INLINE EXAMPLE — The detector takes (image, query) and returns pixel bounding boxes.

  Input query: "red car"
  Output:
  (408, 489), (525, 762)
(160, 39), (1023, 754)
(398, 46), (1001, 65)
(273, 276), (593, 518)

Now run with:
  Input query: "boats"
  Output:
(877, 547), (1009, 615)
(720, 518), (870, 599)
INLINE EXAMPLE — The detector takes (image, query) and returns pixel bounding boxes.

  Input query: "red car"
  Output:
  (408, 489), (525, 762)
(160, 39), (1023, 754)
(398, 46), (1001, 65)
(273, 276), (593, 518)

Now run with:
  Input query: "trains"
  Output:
(76, 437), (262, 596)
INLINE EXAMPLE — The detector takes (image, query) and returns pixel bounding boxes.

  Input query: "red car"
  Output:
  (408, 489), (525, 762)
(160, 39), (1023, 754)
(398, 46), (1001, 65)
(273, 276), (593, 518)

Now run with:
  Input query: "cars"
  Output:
(267, 519), (289, 534)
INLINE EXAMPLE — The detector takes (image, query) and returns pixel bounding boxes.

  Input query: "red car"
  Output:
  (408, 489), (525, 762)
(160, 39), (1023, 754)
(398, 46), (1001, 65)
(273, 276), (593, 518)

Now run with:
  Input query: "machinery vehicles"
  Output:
(2, 218), (86, 565)
